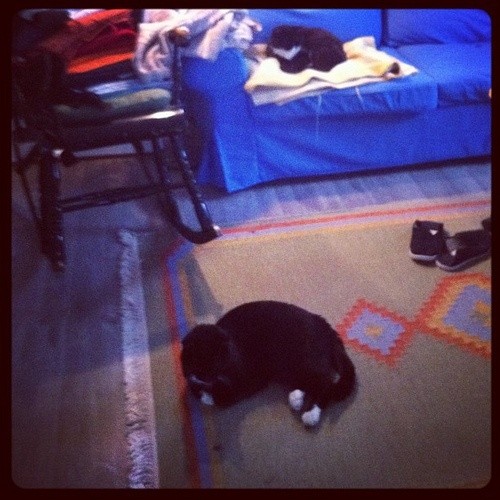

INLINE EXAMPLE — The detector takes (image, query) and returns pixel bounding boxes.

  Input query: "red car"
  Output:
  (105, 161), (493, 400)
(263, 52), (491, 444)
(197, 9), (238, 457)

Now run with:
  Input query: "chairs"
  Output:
(12, 26), (223, 273)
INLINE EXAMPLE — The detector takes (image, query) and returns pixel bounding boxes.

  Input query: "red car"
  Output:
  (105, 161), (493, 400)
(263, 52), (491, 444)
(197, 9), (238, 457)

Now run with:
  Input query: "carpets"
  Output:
(116, 189), (490, 489)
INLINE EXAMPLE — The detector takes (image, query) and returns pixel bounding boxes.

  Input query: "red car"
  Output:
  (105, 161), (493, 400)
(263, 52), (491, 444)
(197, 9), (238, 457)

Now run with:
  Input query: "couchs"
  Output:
(180, 9), (492, 193)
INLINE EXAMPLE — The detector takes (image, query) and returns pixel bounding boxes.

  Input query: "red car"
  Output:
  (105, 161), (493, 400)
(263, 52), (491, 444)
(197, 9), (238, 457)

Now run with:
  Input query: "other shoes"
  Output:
(409, 220), (447, 261)
(436, 229), (491, 271)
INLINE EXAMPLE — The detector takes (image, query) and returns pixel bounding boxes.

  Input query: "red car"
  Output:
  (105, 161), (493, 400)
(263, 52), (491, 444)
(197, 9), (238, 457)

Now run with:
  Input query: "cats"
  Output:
(180, 300), (356, 427)
(265, 25), (347, 74)
